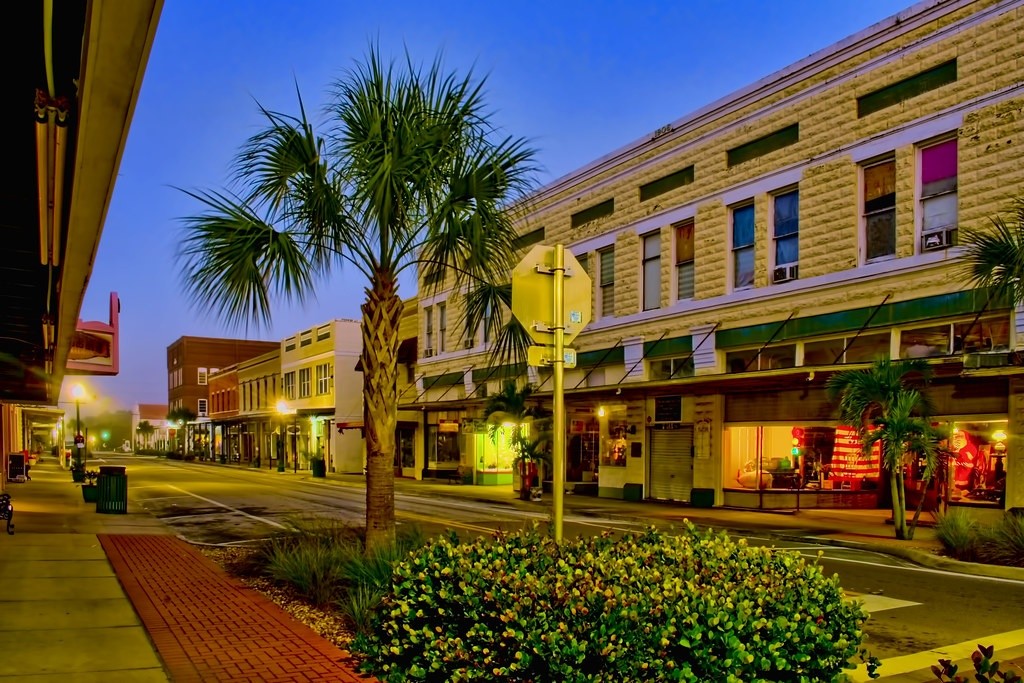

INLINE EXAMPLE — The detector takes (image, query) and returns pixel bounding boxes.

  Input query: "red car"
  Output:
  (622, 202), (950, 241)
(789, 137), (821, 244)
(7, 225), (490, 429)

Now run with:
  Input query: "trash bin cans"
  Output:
(218, 455), (227, 465)
(312, 458), (326, 477)
(95, 465), (127, 514)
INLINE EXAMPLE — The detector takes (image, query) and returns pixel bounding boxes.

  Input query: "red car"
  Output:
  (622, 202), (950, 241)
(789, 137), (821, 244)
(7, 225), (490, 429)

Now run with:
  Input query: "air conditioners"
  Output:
(772, 263), (796, 284)
(921, 225), (953, 251)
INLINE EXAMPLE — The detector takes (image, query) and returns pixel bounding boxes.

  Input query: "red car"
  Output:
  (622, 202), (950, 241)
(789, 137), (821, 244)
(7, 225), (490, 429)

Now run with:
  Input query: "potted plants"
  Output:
(69, 460), (86, 482)
(298, 446), (326, 478)
(82, 471), (99, 503)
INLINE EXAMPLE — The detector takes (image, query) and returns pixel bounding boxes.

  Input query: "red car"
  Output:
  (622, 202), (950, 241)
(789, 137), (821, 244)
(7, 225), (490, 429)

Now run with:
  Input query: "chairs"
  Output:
(0, 493), (15, 535)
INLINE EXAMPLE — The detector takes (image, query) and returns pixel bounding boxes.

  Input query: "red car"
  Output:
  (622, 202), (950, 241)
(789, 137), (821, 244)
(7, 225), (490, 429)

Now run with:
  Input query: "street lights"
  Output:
(276, 401), (287, 472)
(69, 384), (85, 467)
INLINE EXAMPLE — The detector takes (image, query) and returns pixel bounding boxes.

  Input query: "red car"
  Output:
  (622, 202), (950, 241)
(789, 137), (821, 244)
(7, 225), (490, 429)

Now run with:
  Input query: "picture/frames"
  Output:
(570, 419), (586, 435)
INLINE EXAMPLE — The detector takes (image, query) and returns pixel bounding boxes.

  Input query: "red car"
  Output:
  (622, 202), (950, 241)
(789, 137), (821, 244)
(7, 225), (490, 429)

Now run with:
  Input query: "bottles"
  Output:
(781, 456), (790, 468)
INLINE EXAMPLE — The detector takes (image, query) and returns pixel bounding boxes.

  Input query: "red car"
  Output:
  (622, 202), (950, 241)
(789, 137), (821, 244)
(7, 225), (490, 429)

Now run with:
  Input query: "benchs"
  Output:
(448, 466), (473, 484)
